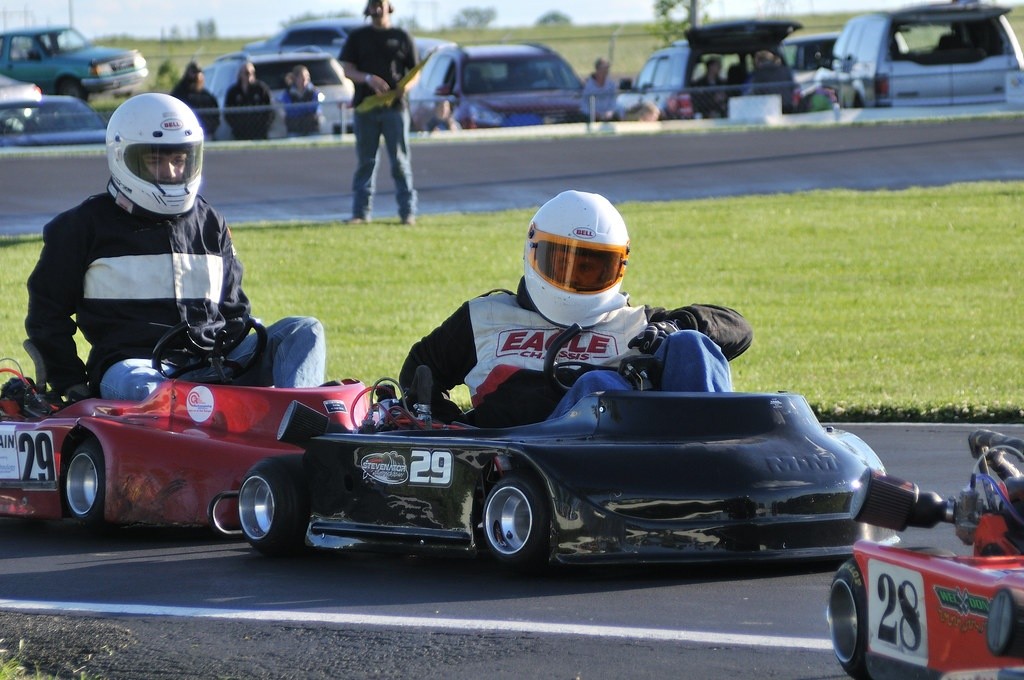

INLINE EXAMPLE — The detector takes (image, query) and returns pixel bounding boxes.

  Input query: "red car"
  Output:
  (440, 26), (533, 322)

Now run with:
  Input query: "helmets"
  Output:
(105, 92), (205, 219)
(523, 189), (633, 329)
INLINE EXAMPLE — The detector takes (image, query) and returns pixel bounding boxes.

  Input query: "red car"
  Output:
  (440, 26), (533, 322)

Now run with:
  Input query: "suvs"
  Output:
(243, 17), (370, 58)
(617, 18), (804, 118)
(816, 3), (1024, 109)
(199, 45), (355, 135)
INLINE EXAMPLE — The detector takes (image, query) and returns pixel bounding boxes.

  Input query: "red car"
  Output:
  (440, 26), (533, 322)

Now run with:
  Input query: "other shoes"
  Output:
(345, 216), (371, 225)
(400, 214), (415, 225)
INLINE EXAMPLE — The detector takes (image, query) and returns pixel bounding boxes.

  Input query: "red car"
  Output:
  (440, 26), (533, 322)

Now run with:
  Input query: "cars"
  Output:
(0, 74), (108, 147)
(782, 29), (912, 103)
(407, 41), (588, 130)
(0, 25), (151, 103)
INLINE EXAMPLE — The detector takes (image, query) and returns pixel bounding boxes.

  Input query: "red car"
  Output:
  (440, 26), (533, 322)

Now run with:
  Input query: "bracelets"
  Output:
(365, 74), (372, 82)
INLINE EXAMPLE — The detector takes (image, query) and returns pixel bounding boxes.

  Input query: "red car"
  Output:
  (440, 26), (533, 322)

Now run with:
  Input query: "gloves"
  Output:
(627, 320), (678, 354)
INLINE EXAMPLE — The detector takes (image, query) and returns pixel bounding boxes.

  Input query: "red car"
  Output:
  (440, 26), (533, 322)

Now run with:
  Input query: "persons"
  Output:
(222, 63), (275, 140)
(338, 0), (419, 224)
(278, 65), (324, 136)
(742, 49), (793, 111)
(175, 65), (220, 139)
(395, 189), (751, 427)
(691, 57), (727, 118)
(427, 99), (462, 131)
(23, 91), (326, 399)
(579, 57), (617, 120)
